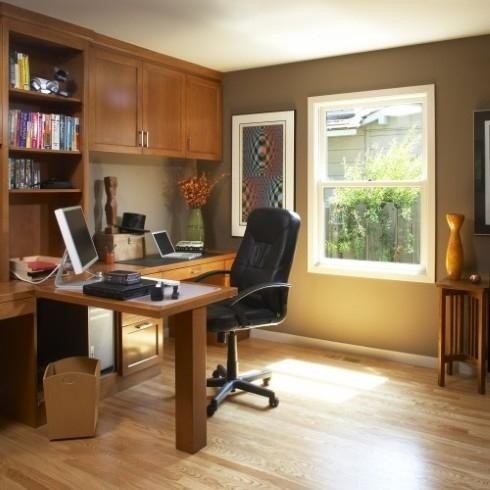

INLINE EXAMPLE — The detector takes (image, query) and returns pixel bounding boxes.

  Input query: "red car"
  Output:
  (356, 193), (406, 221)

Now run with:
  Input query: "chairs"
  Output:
(185, 209), (301, 416)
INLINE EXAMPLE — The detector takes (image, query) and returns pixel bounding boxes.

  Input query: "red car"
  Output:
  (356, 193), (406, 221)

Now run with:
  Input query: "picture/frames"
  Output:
(472, 110), (490, 236)
(229, 109), (296, 238)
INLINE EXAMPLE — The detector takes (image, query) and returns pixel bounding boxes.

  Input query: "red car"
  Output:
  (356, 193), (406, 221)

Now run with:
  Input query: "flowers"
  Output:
(179, 171), (225, 209)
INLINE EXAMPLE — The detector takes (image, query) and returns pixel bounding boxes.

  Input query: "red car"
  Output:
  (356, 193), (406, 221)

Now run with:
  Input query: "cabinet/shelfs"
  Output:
(180, 71), (225, 161)
(89, 46), (185, 160)
(0, 10), (89, 282)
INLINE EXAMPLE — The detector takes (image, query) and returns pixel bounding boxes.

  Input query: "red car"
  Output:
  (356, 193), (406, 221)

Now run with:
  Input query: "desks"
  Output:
(437, 277), (490, 396)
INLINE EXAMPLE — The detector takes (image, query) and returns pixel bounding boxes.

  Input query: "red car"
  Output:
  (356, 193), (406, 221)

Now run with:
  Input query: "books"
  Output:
(9, 52), (81, 191)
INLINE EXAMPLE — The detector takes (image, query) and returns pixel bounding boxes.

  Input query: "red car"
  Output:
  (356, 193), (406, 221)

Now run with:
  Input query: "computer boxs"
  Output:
(38, 298), (115, 376)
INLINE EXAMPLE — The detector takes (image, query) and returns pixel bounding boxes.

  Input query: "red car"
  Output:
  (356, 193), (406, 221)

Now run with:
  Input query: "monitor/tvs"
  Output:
(54, 206), (99, 287)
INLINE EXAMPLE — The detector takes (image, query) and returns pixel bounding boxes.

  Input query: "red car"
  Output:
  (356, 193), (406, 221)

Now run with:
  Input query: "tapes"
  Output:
(156, 281), (162, 288)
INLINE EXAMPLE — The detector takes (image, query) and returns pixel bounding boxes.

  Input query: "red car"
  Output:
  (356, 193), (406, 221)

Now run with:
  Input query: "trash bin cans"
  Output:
(43, 357), (100, 441)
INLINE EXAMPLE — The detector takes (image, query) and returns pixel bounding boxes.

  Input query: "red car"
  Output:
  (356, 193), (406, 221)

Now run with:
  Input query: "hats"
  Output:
(114, 213), (149, 232)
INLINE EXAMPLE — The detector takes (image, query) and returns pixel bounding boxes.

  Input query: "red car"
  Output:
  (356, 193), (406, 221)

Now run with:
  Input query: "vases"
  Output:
(444, 212), (465, 280)
(187, 205), (205, 243)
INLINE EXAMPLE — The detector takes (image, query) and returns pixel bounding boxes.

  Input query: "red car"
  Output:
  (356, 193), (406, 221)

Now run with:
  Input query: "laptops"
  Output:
(152, 230), (202, 260)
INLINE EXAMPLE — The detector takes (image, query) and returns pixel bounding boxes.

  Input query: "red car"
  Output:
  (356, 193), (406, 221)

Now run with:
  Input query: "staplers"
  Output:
(172, 285), (179, 299)
